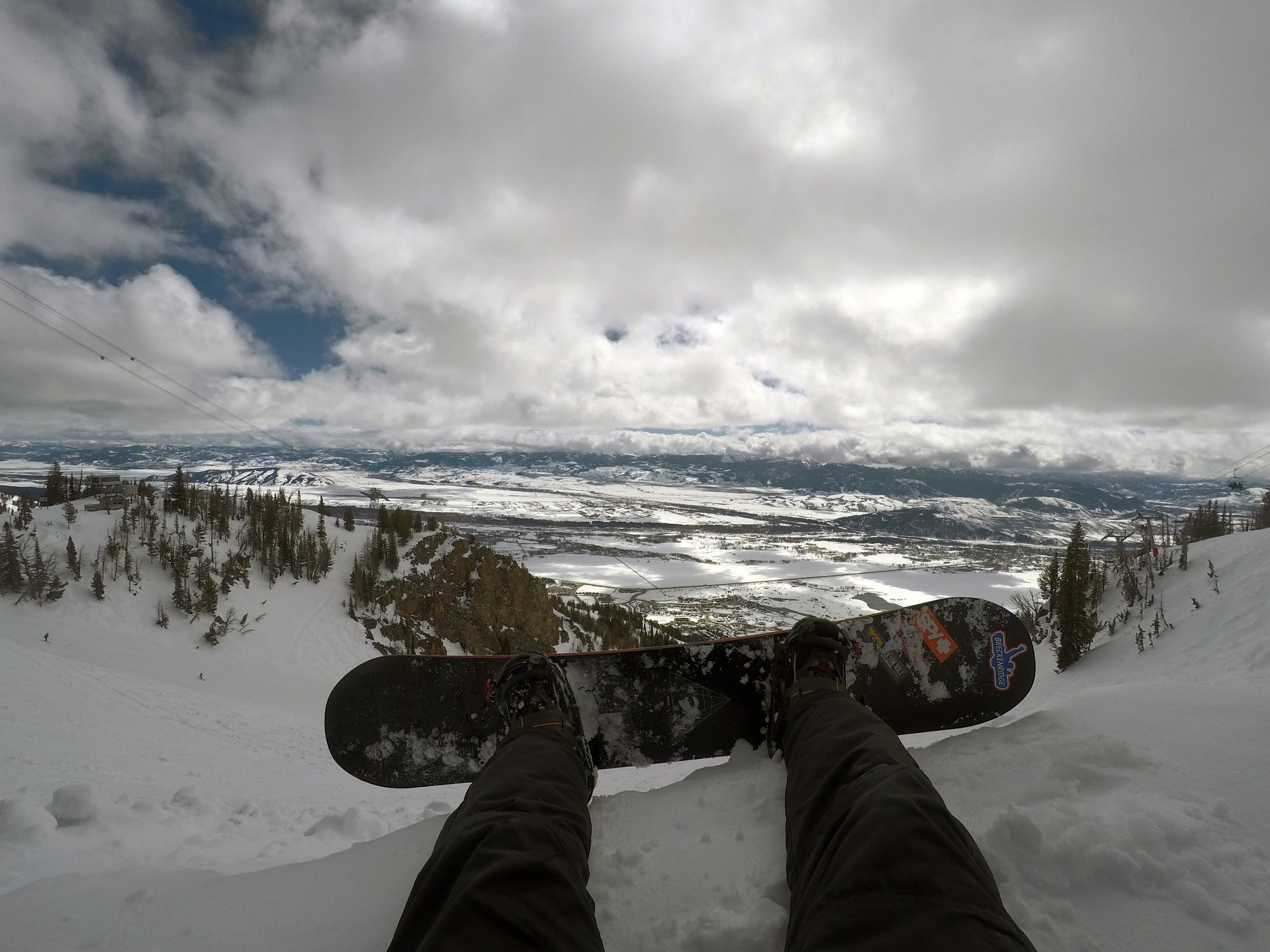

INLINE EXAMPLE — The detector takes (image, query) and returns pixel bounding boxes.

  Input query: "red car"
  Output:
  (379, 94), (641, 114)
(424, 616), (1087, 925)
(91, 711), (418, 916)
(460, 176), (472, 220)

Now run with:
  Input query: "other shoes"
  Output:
(781, 618), (847, 690)
(500, 652), (596, 809)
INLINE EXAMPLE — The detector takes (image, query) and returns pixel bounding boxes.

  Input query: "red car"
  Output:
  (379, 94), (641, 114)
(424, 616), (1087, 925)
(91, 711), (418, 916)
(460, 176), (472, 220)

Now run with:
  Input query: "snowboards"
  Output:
(320, 596), (1038, 791)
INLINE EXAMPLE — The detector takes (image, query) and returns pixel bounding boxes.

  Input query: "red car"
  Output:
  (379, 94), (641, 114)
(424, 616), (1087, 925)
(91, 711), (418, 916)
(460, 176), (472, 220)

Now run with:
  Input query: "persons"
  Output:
(1145, 526), (1147, 531)
(1142, 524), (1145, 531)
(1233, 482), (1237, 490)
(1237, 482), (1243, 490)
(387, 617), (1039, 952)
(1229, 482), (1234, 489)
(1140, 524), (1143, 530)
